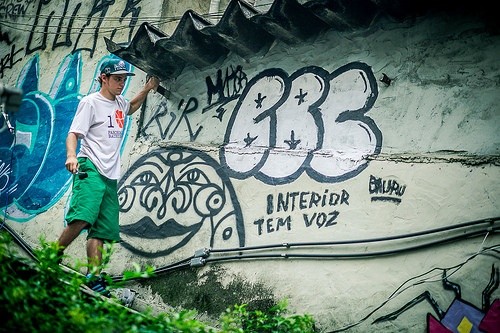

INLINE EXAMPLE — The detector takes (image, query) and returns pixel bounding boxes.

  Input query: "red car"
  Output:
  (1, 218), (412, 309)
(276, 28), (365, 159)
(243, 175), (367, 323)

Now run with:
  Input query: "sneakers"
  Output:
(84, 273), (112, 298)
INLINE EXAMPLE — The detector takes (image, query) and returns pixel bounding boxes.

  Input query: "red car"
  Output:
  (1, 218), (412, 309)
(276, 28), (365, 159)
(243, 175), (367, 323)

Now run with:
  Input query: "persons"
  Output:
(47, 57), (158, 296)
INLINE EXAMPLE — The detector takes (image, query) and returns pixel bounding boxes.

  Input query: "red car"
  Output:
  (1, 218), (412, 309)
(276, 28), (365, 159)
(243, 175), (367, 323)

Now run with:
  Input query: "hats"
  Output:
(100, 59), (136, 77)
